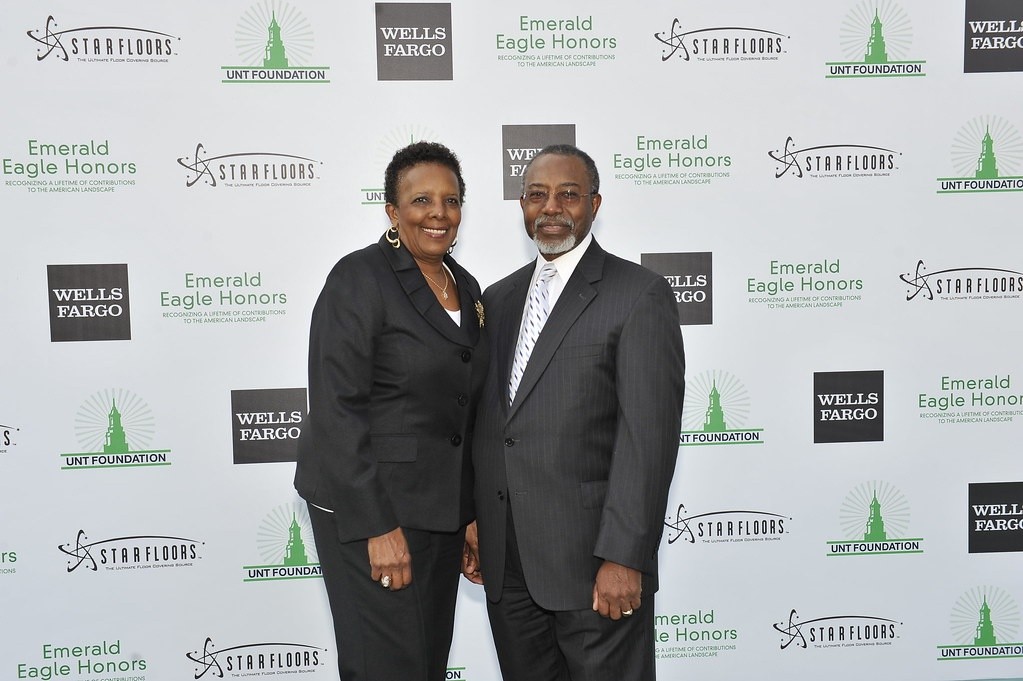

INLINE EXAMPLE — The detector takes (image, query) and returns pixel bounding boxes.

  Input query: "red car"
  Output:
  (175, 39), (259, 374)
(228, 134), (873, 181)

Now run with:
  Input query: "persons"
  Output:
(462, 144), (686, 681)
(291, 140), (481, 680)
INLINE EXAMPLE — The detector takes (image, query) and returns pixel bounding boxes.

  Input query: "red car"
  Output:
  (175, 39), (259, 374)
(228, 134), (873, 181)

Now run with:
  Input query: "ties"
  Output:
(507, 262), (558, 406)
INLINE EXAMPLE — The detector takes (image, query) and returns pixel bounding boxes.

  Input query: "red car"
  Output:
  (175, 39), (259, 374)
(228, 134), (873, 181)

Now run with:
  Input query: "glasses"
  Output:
(521, 189), (593, 205)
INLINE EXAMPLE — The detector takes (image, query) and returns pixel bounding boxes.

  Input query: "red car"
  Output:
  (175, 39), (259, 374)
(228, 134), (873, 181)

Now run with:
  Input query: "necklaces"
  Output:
(421, 265), (449, 299)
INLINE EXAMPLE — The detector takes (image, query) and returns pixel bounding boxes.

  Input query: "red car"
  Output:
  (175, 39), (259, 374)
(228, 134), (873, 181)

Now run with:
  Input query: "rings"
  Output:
(381, 575), (391, 587)
(621, 608), (633, 615)
